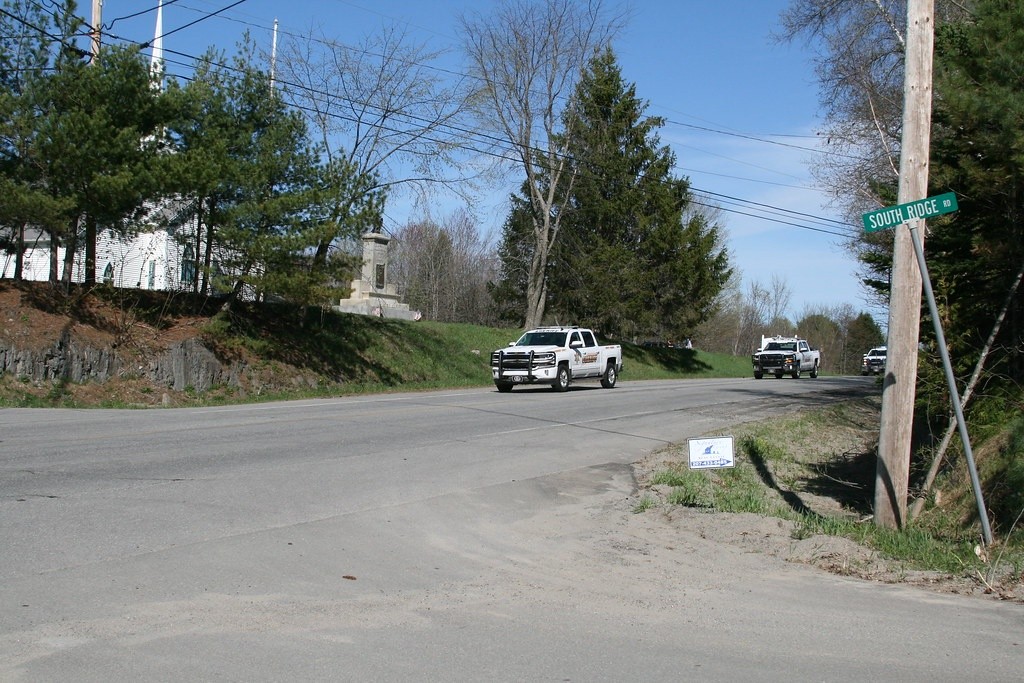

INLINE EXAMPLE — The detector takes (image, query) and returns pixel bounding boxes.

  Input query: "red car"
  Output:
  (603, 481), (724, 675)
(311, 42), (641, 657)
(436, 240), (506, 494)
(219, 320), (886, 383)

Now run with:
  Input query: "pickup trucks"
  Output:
(490, 324), (624, 391)
(861, 347), (887, 376)
(751, 338), (821, 380)
